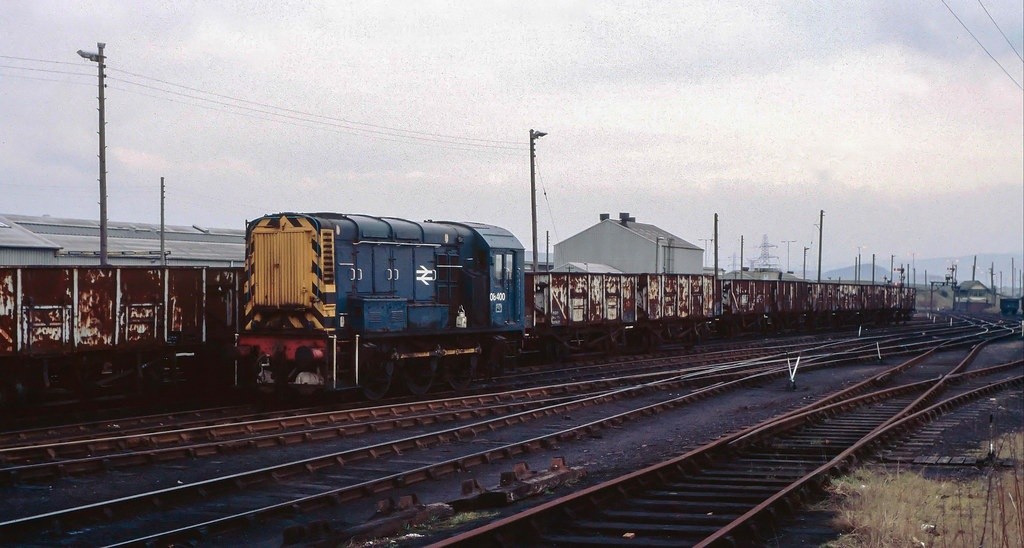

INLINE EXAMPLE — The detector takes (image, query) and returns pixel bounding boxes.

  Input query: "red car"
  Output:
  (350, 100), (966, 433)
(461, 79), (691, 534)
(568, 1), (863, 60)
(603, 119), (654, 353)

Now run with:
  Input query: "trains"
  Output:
(0, 209), (917, 416)
(230, 212), (525, 397)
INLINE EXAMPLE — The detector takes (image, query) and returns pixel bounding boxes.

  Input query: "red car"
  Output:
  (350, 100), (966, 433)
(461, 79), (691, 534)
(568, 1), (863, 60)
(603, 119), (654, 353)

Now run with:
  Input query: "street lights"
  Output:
(530, 131), (546, 272)
(78, 49), (107, 263)
